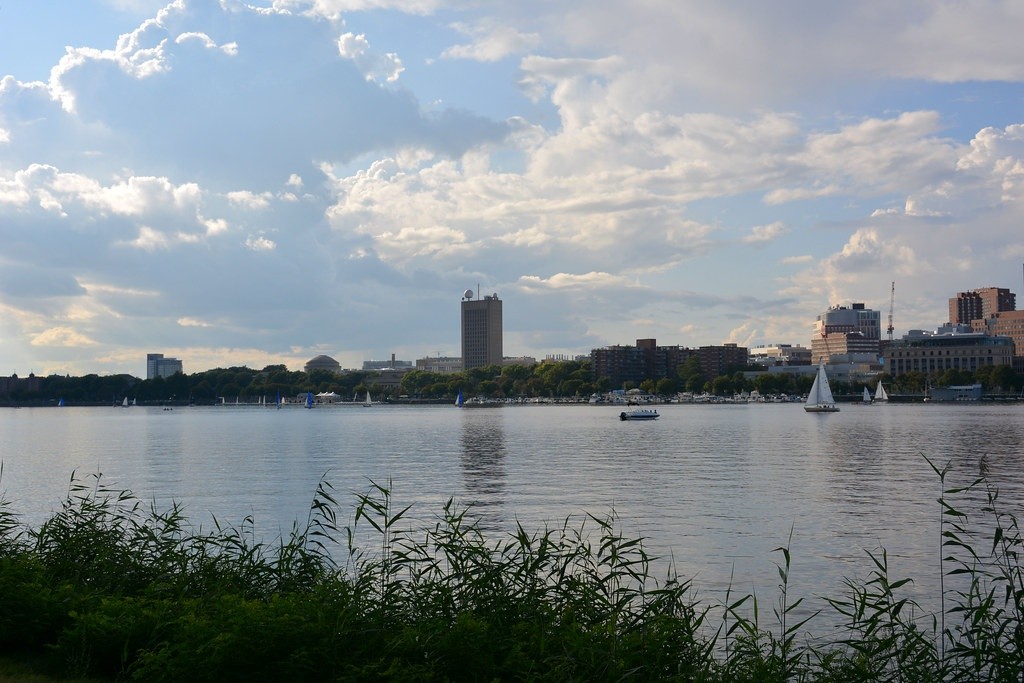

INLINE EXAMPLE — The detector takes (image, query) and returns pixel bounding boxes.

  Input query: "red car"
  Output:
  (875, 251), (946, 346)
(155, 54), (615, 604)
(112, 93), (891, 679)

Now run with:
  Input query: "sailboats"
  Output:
(362, 391), (372, 407)
(121, 396), (129, 407)
(454, 389), (464, 407)
(803, 360), (840, 412)
(303, 389), (316, 408)
(275, 390), (284, 409)
(862, 386), (871, 402)
(872, 380), (888, 402)
(57, 399), (65, 407)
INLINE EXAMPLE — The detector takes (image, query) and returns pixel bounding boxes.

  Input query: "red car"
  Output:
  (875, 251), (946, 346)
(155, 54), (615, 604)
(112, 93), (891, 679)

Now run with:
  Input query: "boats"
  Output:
(924, 396), (944, 404)
(619, 409), (660, 420)
